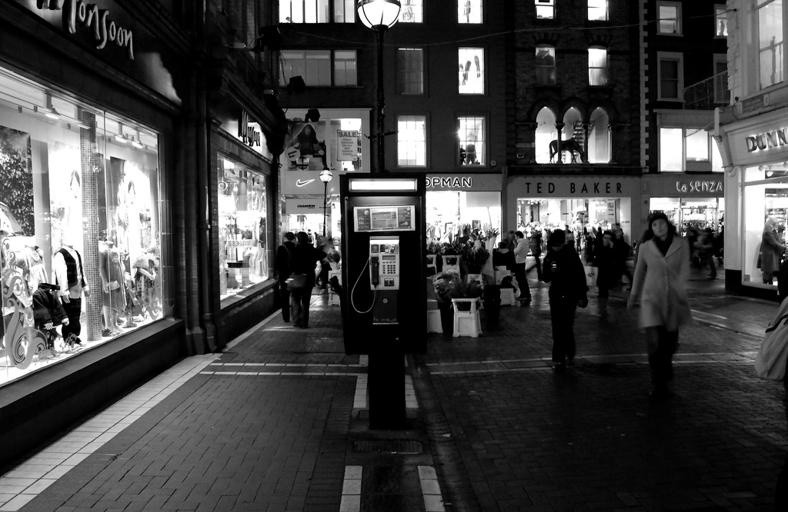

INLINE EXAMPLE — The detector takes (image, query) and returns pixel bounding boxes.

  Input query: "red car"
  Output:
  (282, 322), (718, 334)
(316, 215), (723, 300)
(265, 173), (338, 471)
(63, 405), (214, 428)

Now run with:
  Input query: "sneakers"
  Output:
(515, 294), (532, 302)
(553, 362), (580, 374)
(294, 320), (308, 329)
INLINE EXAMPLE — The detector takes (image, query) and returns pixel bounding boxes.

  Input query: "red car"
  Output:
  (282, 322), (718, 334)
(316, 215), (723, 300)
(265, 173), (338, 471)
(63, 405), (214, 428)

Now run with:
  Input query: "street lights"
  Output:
(317, 166), (336, 244)
(351, 0), (406, 170)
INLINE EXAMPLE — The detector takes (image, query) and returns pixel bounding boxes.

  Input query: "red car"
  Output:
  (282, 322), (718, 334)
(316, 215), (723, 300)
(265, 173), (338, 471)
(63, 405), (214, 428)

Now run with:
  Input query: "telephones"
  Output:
(369, 235), (401, 291)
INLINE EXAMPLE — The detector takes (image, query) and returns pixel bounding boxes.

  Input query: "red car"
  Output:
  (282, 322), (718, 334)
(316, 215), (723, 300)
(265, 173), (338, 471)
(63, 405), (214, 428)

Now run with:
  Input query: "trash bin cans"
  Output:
(227, 262), (250, 290)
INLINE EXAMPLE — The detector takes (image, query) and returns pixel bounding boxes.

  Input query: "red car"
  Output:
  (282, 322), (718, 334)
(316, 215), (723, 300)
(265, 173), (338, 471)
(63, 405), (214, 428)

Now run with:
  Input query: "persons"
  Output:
(286, 230), (316, 327)
(132, 253), (159, 314)
(627, 211), (690, 397)
(499, 222), (724, 369)
(55, 241), (88, 345)
(316, 238), (335, 289)
(289, 124), (328, 171)
(99, 239), (128, 333)
(755, 217), (787, 284)
(33, 282), (70, 356)
(274, 233), (296, 321)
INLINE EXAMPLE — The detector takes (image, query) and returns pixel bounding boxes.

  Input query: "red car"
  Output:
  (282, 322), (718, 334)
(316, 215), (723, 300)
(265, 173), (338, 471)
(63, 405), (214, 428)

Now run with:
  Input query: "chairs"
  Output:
(426, 299), (443, 334)
(549, 119), (596, 166)
(451, 297), (482, 338)
